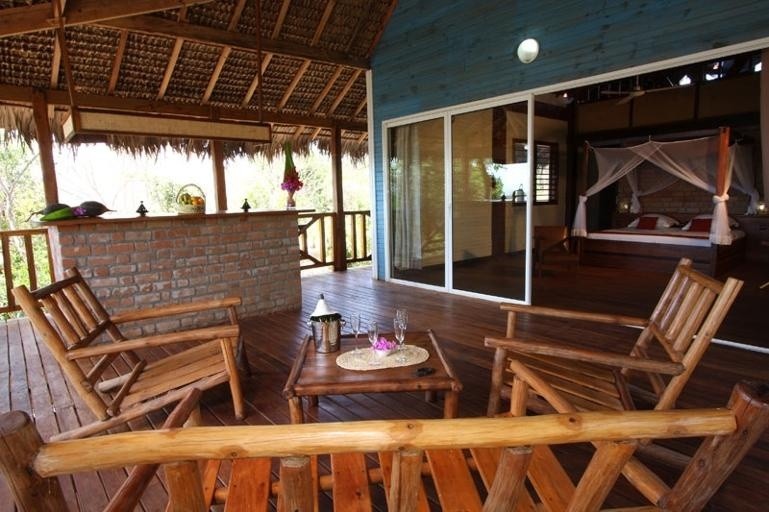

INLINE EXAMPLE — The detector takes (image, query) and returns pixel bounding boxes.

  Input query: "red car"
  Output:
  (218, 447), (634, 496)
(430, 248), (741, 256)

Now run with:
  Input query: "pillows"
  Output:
(627, 212), (739, 233)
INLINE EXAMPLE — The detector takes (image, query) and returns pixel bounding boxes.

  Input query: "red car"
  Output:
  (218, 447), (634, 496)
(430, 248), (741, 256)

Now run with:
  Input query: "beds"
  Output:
(578, 212), (747, 279)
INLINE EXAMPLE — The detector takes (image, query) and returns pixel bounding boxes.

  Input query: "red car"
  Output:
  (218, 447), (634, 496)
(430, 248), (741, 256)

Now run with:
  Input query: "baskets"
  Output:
(174, 184), (208, 215)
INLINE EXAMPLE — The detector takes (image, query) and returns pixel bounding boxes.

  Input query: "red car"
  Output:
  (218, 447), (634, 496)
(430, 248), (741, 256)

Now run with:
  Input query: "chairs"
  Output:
(13, 266), (250, 444)
(481, 256), (745, 468)
(534, 224), (582, 279)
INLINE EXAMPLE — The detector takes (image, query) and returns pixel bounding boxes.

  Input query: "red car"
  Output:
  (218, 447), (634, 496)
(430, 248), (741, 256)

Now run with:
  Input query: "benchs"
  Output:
(1, 379), (768, 512)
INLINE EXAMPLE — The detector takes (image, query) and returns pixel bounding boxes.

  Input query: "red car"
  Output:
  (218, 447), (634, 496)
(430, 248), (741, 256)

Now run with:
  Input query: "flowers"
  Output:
(280, 168), (304, 194)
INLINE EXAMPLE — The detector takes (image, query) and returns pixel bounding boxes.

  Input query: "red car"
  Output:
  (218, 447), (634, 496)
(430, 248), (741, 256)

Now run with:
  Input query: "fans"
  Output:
(601, 75), (675, 109)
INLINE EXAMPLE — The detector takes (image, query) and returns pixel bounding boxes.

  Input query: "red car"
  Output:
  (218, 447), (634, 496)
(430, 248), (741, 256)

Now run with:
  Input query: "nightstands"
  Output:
(737, 215), (769, 264)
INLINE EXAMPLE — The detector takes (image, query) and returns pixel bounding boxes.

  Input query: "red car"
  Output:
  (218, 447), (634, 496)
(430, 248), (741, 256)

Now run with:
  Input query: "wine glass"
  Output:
(350, 312), (360, 354)
(394, 309), (409, 362)
(368, 321), (378, 365)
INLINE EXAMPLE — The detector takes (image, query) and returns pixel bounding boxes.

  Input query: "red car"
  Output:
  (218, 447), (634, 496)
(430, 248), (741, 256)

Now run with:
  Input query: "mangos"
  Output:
(179, 193), (192, 204)
